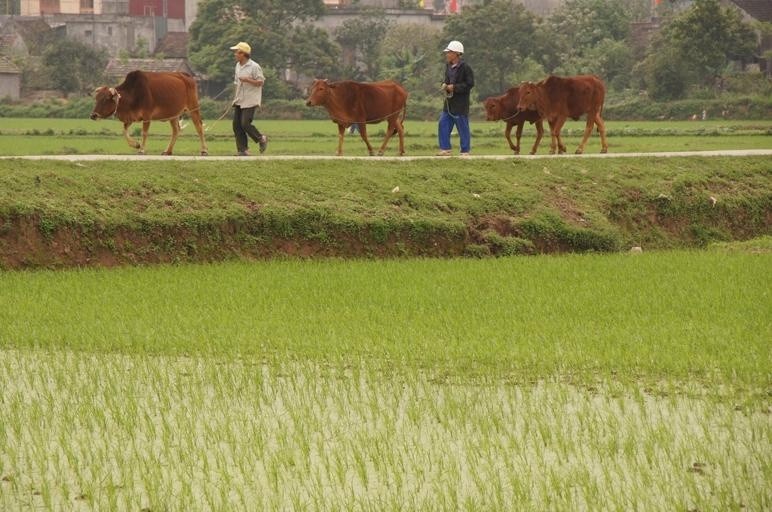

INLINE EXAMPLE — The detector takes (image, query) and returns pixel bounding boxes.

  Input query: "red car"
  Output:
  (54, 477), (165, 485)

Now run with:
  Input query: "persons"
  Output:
(227, 42), (270, 156)
(436, 40), (475, 156)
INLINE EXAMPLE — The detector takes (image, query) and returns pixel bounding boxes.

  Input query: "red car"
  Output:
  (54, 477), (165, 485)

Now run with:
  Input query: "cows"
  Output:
(484, 86), (557, 156)
(510, 74), (610, 155)
(91, 69), (209, 156)
(304, 76), (409, 157)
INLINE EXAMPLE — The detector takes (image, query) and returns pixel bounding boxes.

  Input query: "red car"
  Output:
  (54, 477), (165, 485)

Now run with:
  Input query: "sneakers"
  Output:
(436, 149), (452, 156)
(459, 153), (470, 156)
(259, 135), (268, 153)
(233, 151), (250, 156)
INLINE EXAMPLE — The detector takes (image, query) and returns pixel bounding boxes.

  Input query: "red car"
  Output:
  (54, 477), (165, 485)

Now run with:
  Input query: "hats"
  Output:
(442, 41), (464, 53)
(230, 42), (251, 55)
